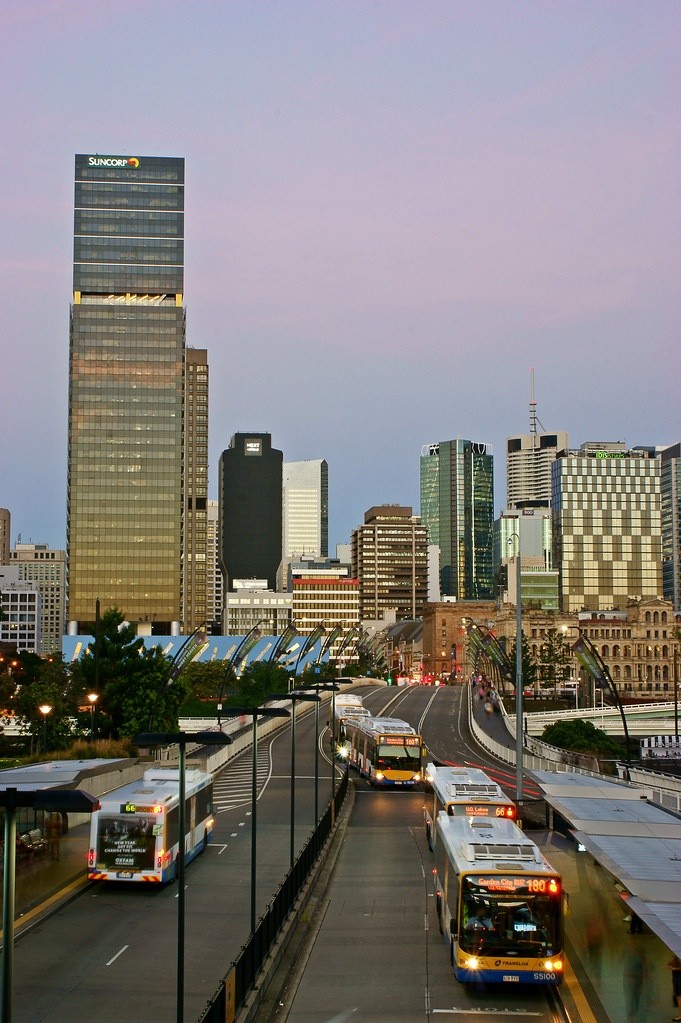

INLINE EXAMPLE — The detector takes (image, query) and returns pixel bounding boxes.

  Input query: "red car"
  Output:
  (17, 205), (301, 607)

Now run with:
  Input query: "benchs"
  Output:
(19, 829), (48, 865)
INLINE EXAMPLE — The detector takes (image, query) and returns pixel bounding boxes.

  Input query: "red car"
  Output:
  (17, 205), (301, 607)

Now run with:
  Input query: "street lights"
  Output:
(462, 615), (527, 716)
(267, 613), (402, 695)
(216, 620), (272, 726)
(39, 698), (54, 754)
(86, 688), (98, 744)
(149, 619), (214, 731)
(559, 622), (631, 766)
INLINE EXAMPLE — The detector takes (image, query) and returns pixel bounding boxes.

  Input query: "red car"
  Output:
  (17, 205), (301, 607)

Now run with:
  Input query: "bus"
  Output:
(423, 760), (522, 858)
(346, 713), (427, 790)
(435, 814), (563, 993)
(330, 693), (360, 749)
(331, 702), (372, 762)
(89, 768), (216, 888)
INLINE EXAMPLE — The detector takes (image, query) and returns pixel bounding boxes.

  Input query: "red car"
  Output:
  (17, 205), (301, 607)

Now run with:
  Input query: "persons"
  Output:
(0, 813), (64, 875)
(667, 954), (681, 1022)
(463, 881), (490, 903)
(509, 888), (551, 942)
(623, 907), (644, 934)
(472, 672), (500, 719)
(103, 817), (148, 868)
(465, 905), (494, 931)
